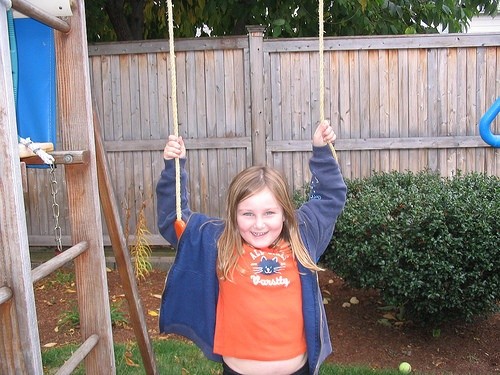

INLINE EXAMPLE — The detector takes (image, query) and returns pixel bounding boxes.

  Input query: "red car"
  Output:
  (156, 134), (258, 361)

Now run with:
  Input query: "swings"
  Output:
(163, 0), (340, 258)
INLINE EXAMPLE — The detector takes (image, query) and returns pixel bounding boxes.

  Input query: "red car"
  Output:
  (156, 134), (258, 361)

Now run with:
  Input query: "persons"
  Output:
(156, 118), (347, 375)
